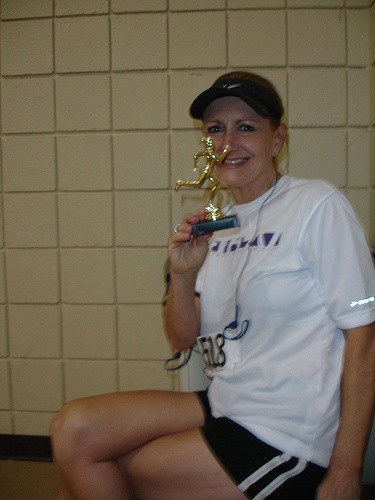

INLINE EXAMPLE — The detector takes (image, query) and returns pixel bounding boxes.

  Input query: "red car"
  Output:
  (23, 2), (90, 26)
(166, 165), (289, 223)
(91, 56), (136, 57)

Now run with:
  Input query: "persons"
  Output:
(48, 70), (375, 499)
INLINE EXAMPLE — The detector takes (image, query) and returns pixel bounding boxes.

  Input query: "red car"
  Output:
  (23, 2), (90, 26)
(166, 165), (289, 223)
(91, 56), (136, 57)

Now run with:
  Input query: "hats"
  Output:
(186, 76), (284, 125)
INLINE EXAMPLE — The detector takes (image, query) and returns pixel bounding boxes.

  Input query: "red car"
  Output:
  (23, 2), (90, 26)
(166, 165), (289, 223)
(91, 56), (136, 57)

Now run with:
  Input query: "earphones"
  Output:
(164, 346), (193, 371)
(222, 320), (249, 340)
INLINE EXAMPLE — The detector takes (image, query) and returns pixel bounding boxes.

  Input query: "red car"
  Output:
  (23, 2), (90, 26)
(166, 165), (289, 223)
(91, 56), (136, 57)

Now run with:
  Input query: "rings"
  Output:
(174, 223), (180, 233)
